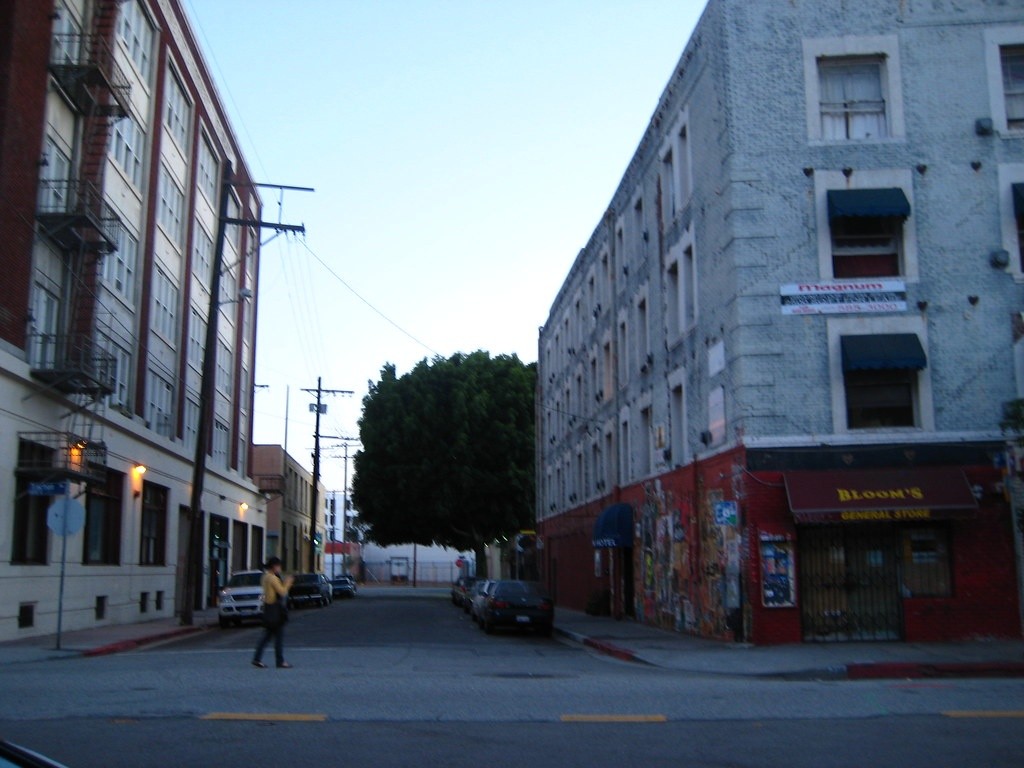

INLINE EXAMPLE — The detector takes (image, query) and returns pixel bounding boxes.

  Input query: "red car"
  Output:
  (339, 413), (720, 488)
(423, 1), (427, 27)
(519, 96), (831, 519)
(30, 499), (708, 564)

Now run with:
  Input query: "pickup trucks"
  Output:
(288, 573), (333, 608)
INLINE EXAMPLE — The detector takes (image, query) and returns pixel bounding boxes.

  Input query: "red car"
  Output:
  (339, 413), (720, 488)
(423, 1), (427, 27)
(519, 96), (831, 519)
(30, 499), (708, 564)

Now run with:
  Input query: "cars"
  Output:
(329, 574), (358, 599)
(478, 580), (556, 637)
(452, 577), (498, 623)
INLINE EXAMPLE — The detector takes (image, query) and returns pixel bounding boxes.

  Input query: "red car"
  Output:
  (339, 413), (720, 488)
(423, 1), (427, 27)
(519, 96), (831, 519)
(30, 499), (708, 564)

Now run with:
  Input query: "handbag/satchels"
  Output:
(262, 603), (288, 628)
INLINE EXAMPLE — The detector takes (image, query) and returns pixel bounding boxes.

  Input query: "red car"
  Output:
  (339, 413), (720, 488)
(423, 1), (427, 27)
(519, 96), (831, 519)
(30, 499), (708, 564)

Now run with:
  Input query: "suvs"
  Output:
(218, 570), (268, 630)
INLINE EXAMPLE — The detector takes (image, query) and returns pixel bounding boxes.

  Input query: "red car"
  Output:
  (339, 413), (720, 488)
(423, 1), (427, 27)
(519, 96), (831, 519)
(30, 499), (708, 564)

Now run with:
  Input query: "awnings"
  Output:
(783, 464), (982, 524)
(841, 333), (929, 373)
(825, 187), (911, 227)
(591, 502), (636, 552)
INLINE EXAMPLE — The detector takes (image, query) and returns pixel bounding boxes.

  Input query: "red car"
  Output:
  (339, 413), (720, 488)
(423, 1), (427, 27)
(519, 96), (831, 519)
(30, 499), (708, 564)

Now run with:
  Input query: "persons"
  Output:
(252, 556), (295, 668)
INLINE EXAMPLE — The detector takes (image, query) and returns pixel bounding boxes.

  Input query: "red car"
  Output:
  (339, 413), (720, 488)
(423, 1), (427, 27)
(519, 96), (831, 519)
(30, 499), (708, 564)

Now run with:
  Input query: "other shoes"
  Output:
(252, 661), (267, 668)
(277, 662), (292, 668)
